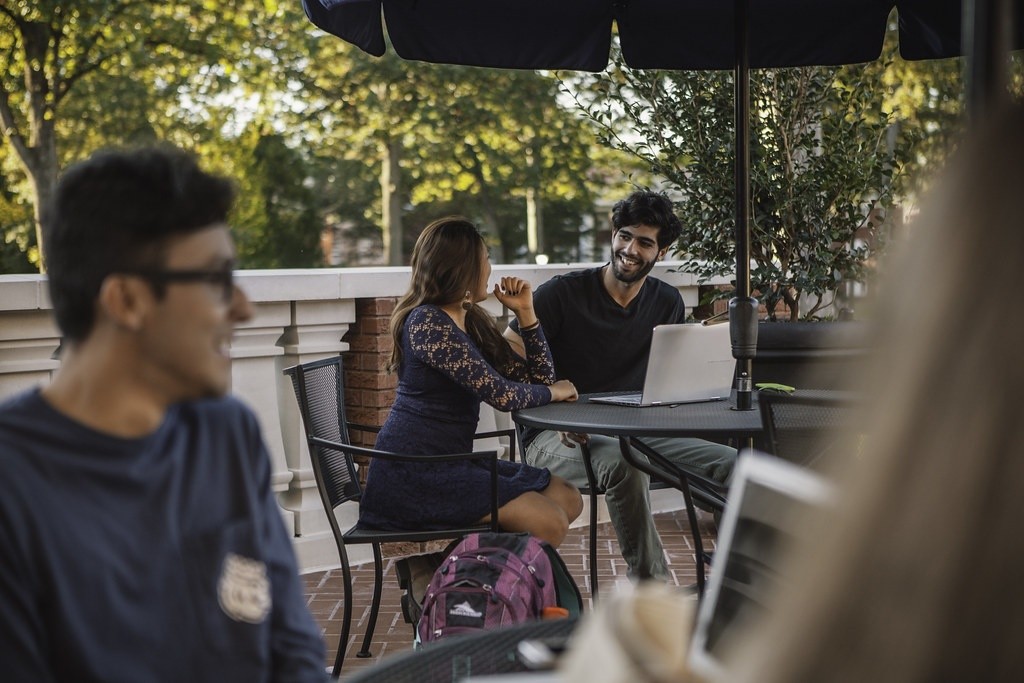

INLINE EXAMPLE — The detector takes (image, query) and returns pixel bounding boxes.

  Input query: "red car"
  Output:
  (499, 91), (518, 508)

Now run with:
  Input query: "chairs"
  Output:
(752, 322), (867, 404)
(514, 423), (677, 609)
(756, 387), (857, 464)
(282, 351), (516, 680)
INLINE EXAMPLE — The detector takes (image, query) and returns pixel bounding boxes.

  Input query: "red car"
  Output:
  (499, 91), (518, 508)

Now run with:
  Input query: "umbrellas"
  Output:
(301, 0), (1024, 413)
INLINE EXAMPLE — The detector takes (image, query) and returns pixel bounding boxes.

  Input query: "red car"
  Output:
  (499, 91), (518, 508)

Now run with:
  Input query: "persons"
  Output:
(749, 97), (1024, 683)
(502, 192), (738, 587)
(0, 142), (329, 683)
(359, 219), (583, 623)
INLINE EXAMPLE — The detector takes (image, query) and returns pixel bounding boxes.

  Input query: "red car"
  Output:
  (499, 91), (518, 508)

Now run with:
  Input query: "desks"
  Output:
(510, 391), (844, 612)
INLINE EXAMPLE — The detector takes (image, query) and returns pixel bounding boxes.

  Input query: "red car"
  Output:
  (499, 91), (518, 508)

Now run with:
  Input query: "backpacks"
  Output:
(412, 530), (584, 650)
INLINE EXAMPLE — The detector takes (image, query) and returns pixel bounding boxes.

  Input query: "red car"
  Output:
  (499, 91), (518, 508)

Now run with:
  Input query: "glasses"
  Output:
(110, 259), (236, 290)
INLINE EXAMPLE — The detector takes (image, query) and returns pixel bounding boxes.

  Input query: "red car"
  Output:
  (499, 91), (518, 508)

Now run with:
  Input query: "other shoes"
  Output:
(394, 554), (440, 624)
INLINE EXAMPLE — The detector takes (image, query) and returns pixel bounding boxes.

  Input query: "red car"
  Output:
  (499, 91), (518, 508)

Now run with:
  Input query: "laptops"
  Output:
(686, 449), (832, 677)
(589, 321), (737, 407)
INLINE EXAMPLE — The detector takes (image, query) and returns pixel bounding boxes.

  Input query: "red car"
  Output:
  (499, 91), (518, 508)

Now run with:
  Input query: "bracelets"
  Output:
(520, 318), (539, 331)
